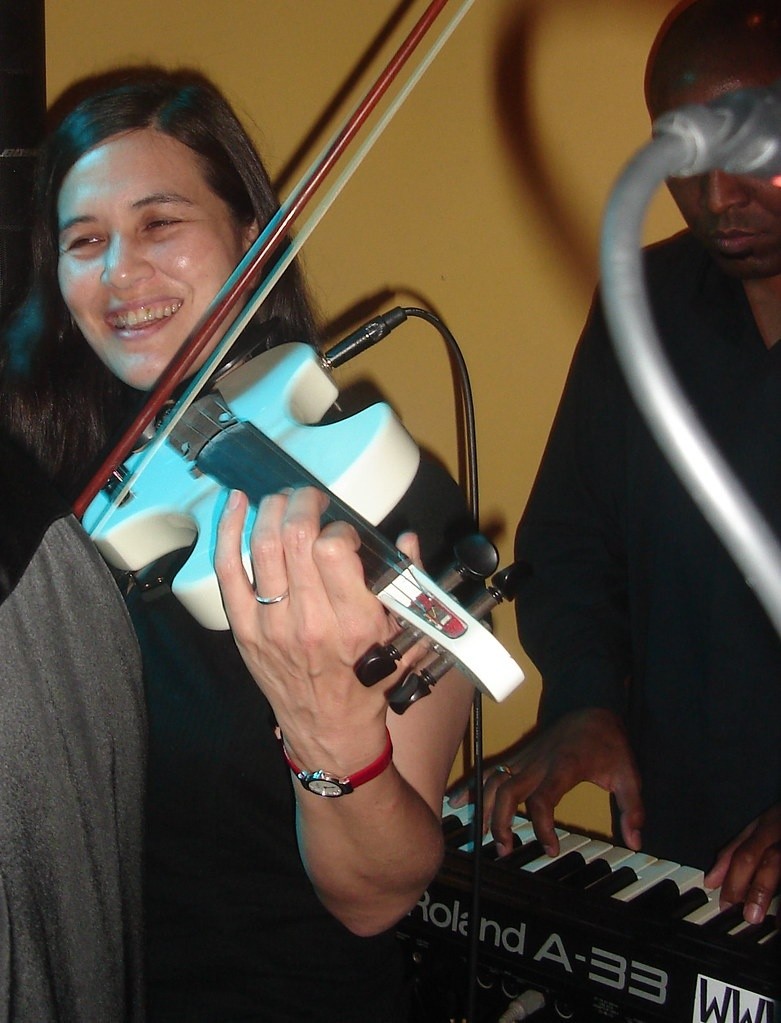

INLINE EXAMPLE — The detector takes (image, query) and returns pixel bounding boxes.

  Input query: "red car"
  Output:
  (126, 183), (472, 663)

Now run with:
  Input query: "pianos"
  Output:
(349, 775), (778, 1023)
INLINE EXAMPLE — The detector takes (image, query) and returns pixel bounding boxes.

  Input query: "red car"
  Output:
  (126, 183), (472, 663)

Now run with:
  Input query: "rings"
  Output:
(254, 589), (289, 605)
(496, 766), (513, 780)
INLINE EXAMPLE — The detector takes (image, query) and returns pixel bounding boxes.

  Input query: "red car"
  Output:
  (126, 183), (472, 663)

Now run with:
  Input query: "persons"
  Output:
(0, 62), (491, 1021)
(443, 0), (781, 924)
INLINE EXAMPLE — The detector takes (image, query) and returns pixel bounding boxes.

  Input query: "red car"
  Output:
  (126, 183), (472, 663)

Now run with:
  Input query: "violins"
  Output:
(74, 338), (533, 714)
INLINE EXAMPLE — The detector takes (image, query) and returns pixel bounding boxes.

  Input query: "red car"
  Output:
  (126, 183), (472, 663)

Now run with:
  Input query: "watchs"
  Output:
(274, 722), (393, 799)
